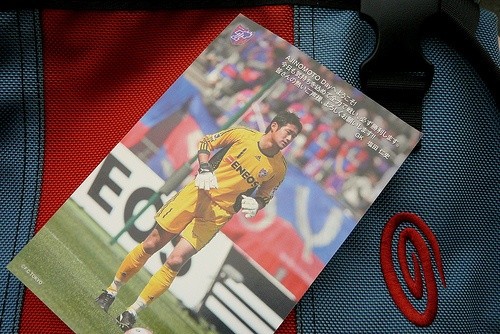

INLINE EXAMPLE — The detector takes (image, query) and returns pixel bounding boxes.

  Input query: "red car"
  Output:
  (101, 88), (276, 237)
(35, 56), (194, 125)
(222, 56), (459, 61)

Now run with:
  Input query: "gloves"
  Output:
(241, 194), (259, 218)
(195, 162), (218, 190)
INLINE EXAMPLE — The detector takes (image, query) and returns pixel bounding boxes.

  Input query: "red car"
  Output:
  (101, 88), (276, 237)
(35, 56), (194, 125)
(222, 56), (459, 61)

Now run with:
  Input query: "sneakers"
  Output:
(115, 311), (136, 333)
(96, 289), (115, 313)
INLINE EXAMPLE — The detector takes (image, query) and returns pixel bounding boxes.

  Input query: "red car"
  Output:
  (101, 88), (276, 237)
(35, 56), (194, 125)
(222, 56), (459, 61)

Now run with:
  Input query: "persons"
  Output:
(94, 110), (303, 331)
